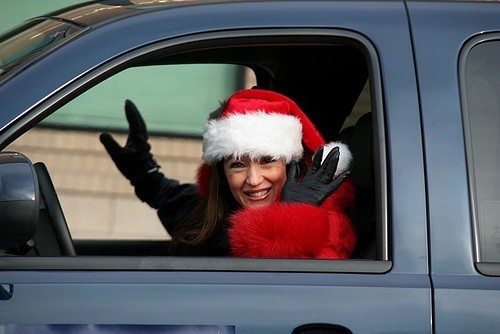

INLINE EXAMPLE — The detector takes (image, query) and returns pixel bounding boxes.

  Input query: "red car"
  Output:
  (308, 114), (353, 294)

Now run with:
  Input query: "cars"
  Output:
(0, 1), (500, 334)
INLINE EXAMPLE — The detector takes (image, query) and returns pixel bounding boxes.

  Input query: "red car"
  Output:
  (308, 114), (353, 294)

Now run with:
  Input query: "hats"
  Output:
(200, 89), (352, 177)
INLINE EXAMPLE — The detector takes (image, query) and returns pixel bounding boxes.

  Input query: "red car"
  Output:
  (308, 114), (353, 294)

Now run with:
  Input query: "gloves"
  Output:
(100, 99), (179, 210)
(279, 146), (350, 207)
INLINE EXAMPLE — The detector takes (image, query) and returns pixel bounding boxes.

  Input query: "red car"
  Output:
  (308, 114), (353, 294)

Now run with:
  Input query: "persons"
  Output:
(99, 86), (261, 257)
(196, 89), (356, 260)
